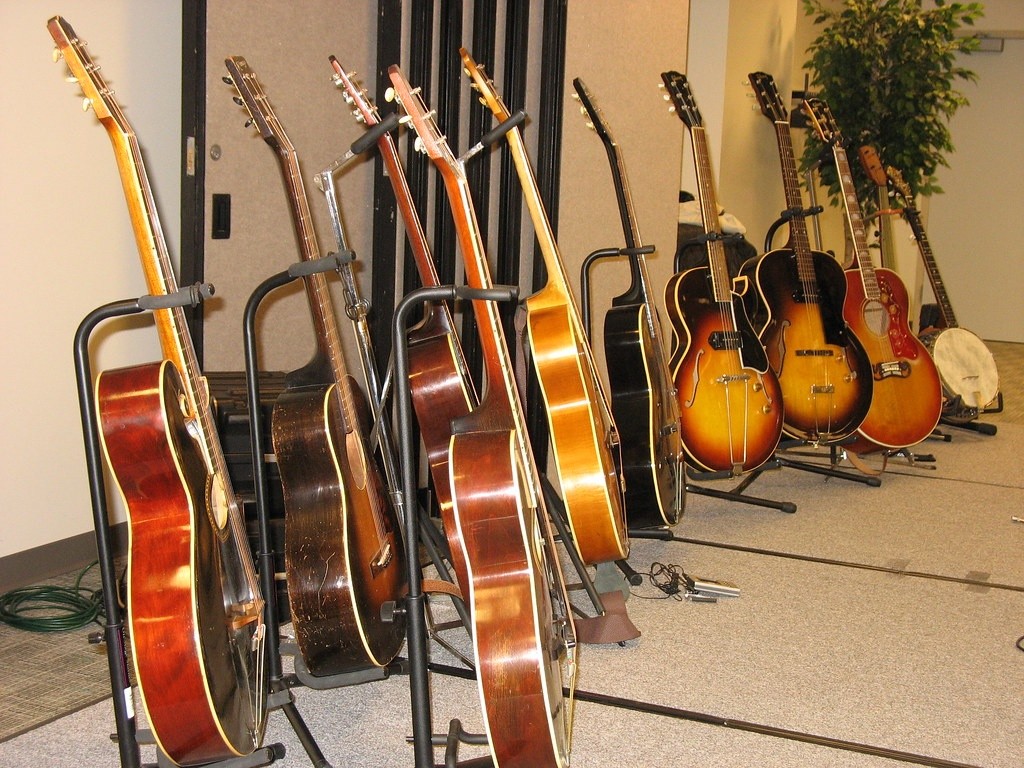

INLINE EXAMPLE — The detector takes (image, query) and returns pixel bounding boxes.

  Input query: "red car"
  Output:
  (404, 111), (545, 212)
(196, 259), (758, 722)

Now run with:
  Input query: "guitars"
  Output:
(740, 71), (875, 446)
(328, 53), (483, 607)
(219, 48), (409, 680)
(885, 166), (999, 412)
(459, 45), (632, 567)
(45, 13), (272, 768)
(658, 70), (786, 478)
(799, 97), (945, 454)
(380, 62), (579, 768)
(571, 75), (688, 530)
